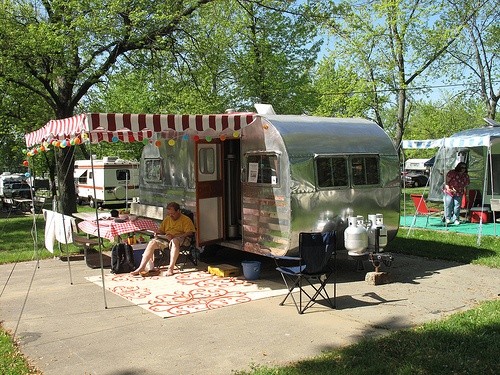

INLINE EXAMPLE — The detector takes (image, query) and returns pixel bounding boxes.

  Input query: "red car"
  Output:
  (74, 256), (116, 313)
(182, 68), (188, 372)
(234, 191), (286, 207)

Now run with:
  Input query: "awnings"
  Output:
(402, 135), (500, 232)
(25, 113), (256, 309)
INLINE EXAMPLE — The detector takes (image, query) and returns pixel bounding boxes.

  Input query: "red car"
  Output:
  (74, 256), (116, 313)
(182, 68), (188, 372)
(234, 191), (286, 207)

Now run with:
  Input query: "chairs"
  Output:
(409, 193), (444, 228)
(157, 226), (199, 271)
(451, 188), (491, 224)
(272, 230), (337, 314)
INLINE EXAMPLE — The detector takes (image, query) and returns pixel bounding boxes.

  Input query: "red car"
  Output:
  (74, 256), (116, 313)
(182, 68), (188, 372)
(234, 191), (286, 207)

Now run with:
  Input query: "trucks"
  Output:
(73, 154), (140, 209)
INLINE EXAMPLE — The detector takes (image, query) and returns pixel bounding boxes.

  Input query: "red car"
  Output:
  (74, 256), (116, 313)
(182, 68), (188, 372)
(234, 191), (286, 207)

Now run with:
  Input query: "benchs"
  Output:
(59, 213), (112, 269)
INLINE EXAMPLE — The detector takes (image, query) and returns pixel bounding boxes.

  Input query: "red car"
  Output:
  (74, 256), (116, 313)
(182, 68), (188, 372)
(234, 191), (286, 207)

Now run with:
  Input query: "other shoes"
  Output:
(454, 220), (460, 225)
(446, 218), (452, 224)
(139, 269), (160, 277)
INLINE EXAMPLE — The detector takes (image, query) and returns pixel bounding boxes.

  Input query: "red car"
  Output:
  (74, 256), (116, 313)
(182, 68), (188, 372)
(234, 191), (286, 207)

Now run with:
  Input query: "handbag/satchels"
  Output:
(461, 194), (467, 209)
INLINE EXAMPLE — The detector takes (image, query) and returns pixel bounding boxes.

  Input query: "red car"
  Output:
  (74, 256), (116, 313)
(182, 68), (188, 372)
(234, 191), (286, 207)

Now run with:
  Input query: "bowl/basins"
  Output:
(110, 209), (139, 222)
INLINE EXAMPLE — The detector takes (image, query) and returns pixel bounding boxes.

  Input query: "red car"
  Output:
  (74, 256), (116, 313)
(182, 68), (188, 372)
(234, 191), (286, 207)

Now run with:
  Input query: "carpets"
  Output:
(85, 261), (301, 319)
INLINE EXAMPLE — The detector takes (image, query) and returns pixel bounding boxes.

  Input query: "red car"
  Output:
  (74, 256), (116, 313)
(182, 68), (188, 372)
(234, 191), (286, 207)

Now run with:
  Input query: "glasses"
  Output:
(461, 167), (466, 169)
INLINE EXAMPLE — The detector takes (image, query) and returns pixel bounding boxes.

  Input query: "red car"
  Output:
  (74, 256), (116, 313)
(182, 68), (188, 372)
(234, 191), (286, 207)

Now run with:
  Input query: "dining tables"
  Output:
(78, 214), (159, 268)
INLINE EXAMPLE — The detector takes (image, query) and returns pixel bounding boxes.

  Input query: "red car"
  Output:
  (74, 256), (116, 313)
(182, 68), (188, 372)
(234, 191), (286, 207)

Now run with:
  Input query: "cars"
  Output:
(0, 172), (59, 215)
(401, 158), (433, 189)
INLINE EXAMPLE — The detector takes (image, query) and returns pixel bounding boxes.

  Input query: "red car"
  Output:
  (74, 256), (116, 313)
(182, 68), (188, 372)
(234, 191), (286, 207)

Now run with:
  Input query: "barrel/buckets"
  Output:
(241, 260), (262, 280)
(130, 243), (149, 269)
(227, 225), (239, 238)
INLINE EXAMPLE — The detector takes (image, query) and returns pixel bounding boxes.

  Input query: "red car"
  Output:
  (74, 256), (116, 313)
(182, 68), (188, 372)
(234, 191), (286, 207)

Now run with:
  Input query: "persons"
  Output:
(130, 202), (196, 276)
(442, 162), (470, 225)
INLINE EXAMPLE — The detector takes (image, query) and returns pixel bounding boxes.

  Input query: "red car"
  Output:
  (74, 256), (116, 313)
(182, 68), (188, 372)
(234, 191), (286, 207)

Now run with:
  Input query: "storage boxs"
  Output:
(208, 264), (240, 277)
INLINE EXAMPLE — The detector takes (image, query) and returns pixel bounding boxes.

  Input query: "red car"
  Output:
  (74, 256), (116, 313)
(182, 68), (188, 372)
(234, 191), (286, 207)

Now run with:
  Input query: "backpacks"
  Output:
(111, 243), (134, 274)
